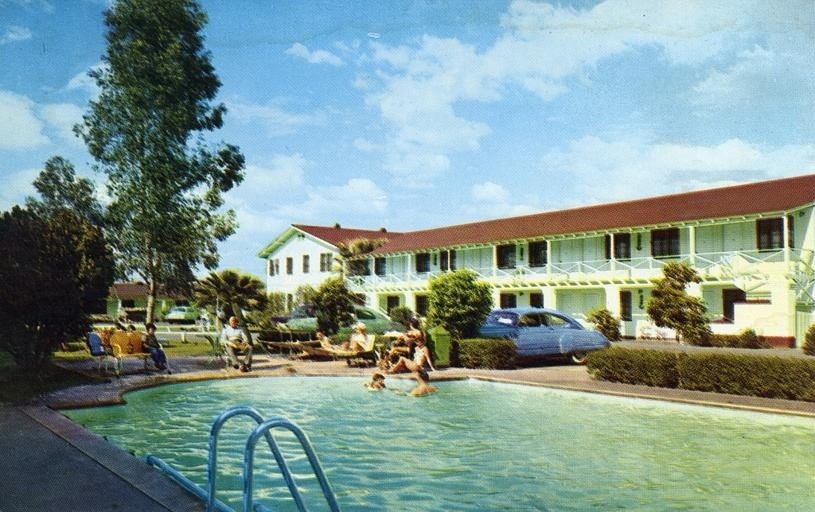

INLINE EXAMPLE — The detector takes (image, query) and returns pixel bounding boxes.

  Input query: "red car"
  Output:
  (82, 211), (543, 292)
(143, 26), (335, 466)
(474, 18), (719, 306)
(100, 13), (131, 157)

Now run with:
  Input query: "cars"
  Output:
(479, 306), (609, 366)
(288, 305), (407, 340)
(165, 306), (199, 325)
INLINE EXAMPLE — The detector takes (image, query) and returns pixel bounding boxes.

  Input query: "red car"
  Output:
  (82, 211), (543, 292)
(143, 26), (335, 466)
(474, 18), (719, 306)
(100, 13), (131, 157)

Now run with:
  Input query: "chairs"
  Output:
(87, 330), (163, 379)
(335, 333), (377, 368)
(228, 352), (251, 369)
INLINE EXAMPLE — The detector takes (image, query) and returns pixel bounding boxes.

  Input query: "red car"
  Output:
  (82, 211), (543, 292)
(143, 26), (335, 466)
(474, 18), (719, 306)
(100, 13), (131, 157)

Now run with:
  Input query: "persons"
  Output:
(369, 317), (439, 396)
(141, 323), (167, 370)
(220, 316), (253, 370)
(340, 321), (367, 352)
(114, 321), (136, 332)
(315, 329), (331, 346)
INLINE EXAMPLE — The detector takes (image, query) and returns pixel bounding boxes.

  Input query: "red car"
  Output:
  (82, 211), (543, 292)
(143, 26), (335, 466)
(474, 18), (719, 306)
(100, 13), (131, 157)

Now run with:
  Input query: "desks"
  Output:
(195, 332), (226, 367)
